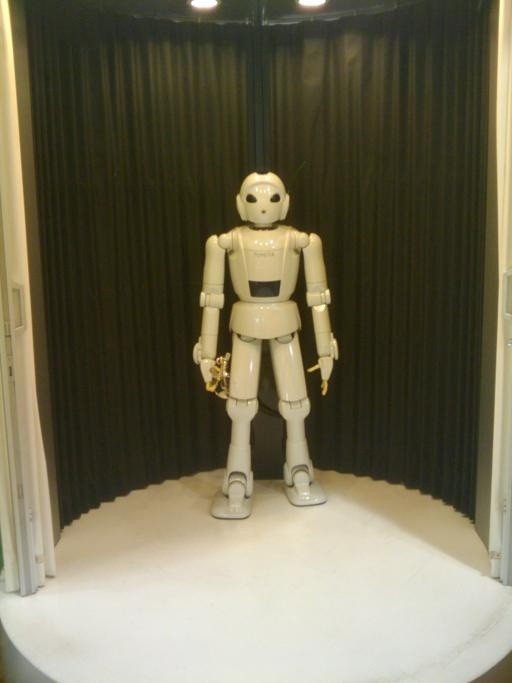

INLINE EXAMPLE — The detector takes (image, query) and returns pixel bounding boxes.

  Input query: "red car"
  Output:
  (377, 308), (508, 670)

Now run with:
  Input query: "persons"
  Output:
(191, 172), (339, 520)
